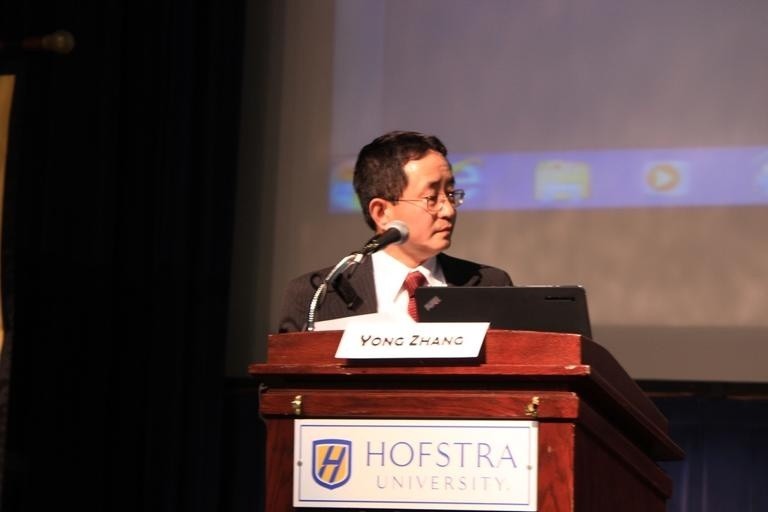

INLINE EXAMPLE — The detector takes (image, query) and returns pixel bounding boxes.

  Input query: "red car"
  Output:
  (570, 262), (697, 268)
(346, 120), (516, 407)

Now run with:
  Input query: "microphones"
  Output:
(357, 219), (410, 255)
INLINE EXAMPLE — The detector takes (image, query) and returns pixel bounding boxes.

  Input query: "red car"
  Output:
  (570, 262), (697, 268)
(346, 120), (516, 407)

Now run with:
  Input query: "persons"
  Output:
(277, 133), (513, 332)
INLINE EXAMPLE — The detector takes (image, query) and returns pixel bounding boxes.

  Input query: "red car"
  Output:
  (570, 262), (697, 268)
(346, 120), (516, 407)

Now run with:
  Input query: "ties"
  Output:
(403, 268), (432, 323)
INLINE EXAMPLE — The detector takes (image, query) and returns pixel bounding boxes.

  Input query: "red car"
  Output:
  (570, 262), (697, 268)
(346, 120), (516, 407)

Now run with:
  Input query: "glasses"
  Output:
(386, 187), (468, 213)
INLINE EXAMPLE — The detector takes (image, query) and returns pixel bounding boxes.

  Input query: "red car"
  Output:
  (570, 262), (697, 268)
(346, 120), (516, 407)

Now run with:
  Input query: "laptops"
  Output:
(413, 285), (594, 340)
